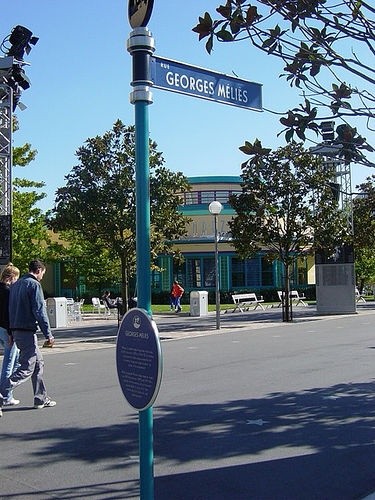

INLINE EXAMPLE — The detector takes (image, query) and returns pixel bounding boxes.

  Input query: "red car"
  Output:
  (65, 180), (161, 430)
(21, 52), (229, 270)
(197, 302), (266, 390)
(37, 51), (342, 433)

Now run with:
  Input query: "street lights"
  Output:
(208, 201), (229, 332)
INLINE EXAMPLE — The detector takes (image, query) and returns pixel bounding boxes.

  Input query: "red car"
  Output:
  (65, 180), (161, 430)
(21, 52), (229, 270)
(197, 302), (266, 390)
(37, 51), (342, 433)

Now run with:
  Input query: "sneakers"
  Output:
(2, 398), (20, 405)
(0, 407), (3, 417)
(34, 399), (56, 408)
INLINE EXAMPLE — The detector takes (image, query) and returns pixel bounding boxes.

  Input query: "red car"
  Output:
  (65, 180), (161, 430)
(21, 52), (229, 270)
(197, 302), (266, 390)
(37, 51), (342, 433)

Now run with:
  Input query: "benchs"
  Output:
(354, 288), (367, 303)
(231, 292), (265, 314)
(277, 290), (307, 308)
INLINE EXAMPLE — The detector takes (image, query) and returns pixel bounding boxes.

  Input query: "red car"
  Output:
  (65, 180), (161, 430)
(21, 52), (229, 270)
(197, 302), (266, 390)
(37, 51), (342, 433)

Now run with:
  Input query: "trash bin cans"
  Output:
(47, 297), (67, 328)
(190, 290), (208, 317)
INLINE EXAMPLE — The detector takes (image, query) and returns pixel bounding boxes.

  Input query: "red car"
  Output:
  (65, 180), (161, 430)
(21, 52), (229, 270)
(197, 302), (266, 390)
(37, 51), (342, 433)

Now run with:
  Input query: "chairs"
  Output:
(103, 302), (117, 316)
(91, 297), (105, 316)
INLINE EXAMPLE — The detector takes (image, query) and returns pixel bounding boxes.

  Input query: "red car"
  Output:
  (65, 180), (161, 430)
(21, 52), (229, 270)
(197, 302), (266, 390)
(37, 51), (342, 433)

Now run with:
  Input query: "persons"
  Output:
(0, 262), (21, 406)
(169, 291), (177, 312)
(102, 290), (123, 309)
(0, 260), (56, 417)
(172, 280), (184, 313)
